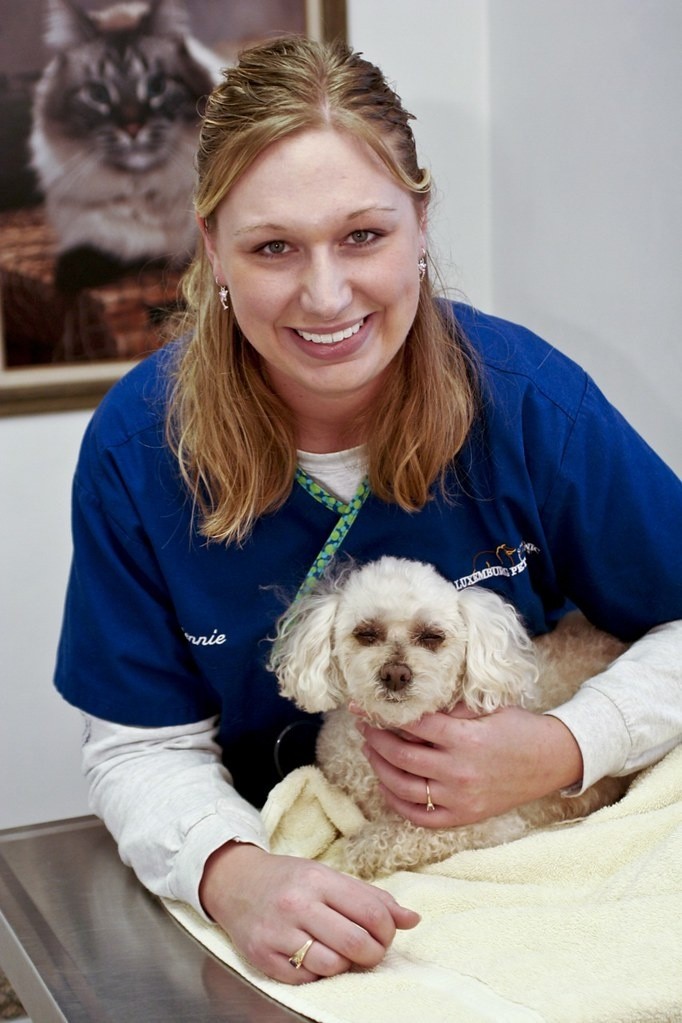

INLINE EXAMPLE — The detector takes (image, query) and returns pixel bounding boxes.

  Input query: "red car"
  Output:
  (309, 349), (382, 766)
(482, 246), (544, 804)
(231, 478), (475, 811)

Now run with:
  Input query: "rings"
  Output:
(426, 778), (435, 812)
(288, 938), (315, 969)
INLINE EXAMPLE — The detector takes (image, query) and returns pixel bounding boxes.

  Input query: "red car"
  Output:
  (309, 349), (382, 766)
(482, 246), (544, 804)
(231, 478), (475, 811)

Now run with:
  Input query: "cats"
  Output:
(25, 0), (242, 299)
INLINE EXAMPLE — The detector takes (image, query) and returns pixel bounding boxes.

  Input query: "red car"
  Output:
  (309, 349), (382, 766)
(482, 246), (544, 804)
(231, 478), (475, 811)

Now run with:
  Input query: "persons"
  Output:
(52, 32), (682, 985)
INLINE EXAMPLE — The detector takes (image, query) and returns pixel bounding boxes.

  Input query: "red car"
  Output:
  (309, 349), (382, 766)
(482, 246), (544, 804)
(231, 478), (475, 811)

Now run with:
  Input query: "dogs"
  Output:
(262, 554), (642, 883)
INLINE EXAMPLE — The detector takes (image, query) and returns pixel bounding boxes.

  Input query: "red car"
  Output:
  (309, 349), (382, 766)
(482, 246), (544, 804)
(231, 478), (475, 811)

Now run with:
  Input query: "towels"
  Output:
(158, 741), (681, 1023)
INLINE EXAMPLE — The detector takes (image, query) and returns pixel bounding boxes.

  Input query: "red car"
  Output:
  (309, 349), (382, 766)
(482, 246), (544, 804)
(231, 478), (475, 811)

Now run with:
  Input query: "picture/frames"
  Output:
(0, 0), (350, 419)
